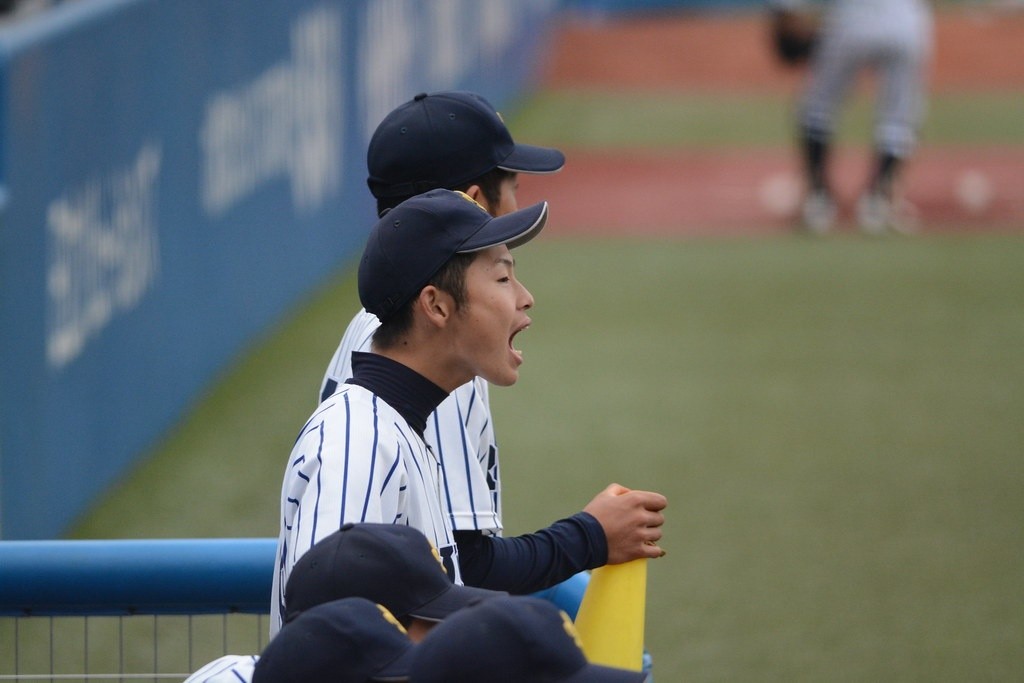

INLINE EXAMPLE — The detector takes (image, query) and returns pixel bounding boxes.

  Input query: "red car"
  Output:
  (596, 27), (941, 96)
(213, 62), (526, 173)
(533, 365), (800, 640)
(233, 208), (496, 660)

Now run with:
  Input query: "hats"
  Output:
(412, 596), (649, 683)
(283, 523), (508, 625)
(358, 189), (549, 322)
(252, 597), (420, 683)
(367, 90), (566, 203)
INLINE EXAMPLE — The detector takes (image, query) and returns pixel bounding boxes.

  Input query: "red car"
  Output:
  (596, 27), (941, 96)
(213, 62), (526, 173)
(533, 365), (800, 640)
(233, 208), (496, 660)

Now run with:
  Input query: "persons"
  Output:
(769, 0), (938, 238)
(266, 188), (537, 654)
(182, 521), (595, 683)
(315, 88), (668, 598)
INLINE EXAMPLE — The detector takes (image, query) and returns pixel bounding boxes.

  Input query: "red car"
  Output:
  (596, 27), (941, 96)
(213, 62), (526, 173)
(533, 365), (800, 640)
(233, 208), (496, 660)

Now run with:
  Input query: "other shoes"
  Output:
(852, 190), (895, 234)
(800, 190), (839, 235)
